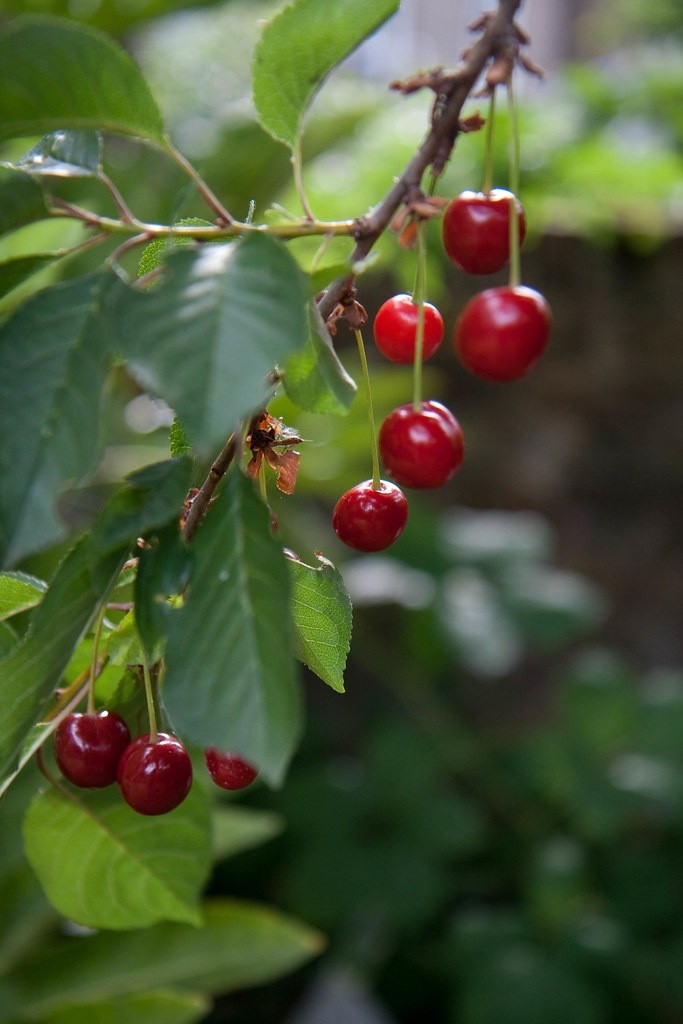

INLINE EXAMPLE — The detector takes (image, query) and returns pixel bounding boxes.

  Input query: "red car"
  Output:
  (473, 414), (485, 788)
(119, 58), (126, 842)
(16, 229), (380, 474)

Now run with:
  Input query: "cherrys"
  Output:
(52, 68), (550, 817)
